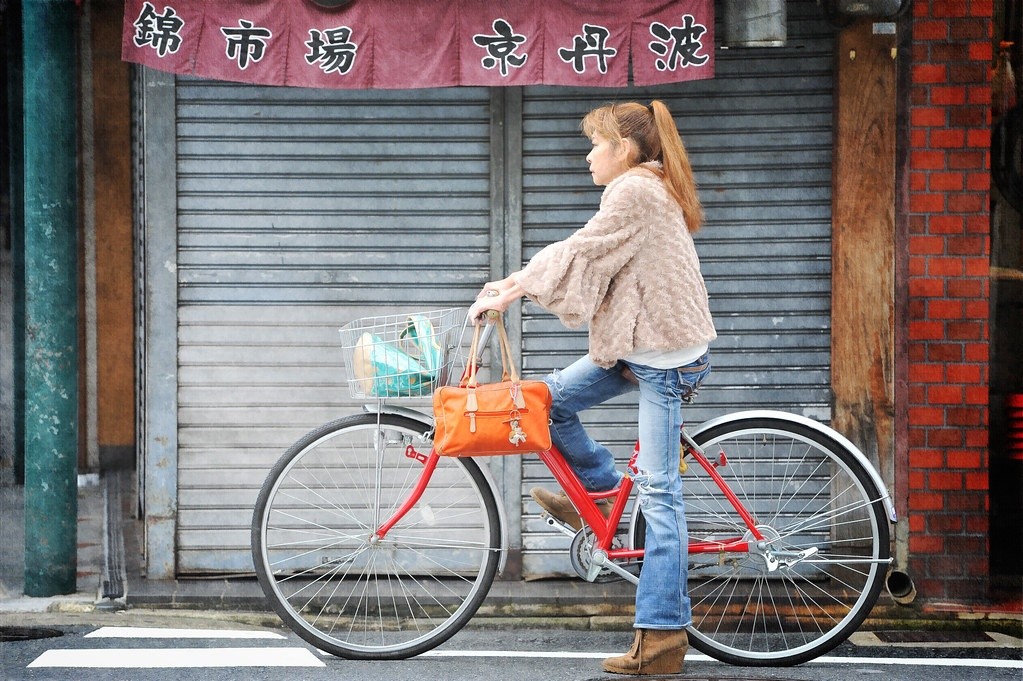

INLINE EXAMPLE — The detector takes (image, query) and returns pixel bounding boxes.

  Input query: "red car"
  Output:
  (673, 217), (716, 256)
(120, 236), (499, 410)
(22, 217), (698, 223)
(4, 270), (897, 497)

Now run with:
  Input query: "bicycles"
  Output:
(247, 289), (899, 667)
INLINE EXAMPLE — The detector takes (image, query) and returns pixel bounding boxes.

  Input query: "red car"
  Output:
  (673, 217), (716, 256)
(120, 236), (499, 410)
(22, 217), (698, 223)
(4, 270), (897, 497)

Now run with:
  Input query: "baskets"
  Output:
(338, 307), (460, 400)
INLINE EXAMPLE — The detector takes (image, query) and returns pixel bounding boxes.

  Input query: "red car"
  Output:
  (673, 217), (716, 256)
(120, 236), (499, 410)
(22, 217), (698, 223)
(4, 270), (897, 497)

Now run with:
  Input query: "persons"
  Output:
(469, 99), (716, 677)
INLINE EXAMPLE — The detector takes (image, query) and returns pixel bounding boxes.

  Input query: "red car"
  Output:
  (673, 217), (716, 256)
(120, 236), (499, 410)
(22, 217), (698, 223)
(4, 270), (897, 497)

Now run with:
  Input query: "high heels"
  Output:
(602, 629), (688, 675)
(530, 487), (617, 532)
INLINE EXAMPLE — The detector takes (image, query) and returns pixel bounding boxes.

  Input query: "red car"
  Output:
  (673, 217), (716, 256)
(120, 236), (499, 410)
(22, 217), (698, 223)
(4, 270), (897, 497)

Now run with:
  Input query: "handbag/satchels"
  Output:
(433, 310), (552, 457)
(353, 316), (446, 396)
